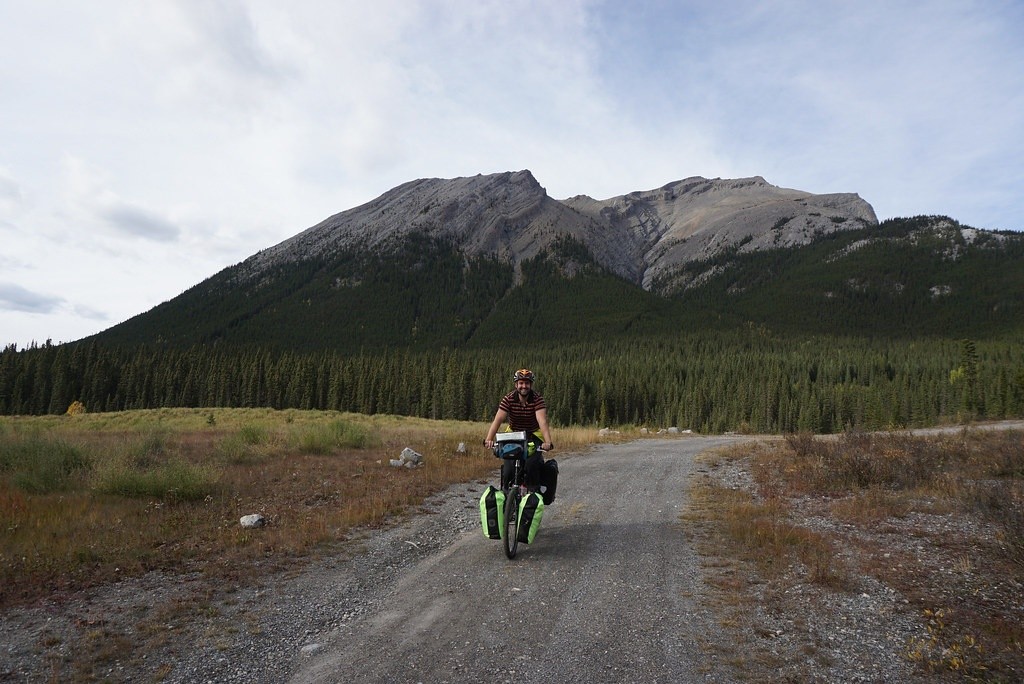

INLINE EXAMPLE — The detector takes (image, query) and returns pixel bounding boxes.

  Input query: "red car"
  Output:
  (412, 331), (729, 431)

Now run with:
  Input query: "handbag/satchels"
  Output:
(542, 459), (558, 505)
(515, 488), (544, 545)
(480, 486), (507, 540)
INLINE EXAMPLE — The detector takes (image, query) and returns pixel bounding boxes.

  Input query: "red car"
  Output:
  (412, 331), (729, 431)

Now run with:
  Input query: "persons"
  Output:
(485, 370), (552, 492)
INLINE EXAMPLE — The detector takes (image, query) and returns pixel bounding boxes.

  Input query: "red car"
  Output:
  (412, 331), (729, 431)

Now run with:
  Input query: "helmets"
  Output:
(514, 369), (535, 383)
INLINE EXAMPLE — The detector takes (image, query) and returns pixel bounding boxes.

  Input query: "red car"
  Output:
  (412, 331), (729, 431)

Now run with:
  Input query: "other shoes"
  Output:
(521, 485), (528, 497)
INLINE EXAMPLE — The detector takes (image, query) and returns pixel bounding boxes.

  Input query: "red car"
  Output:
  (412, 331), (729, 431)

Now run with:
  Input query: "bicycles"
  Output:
(480, 438), (553, 559)
